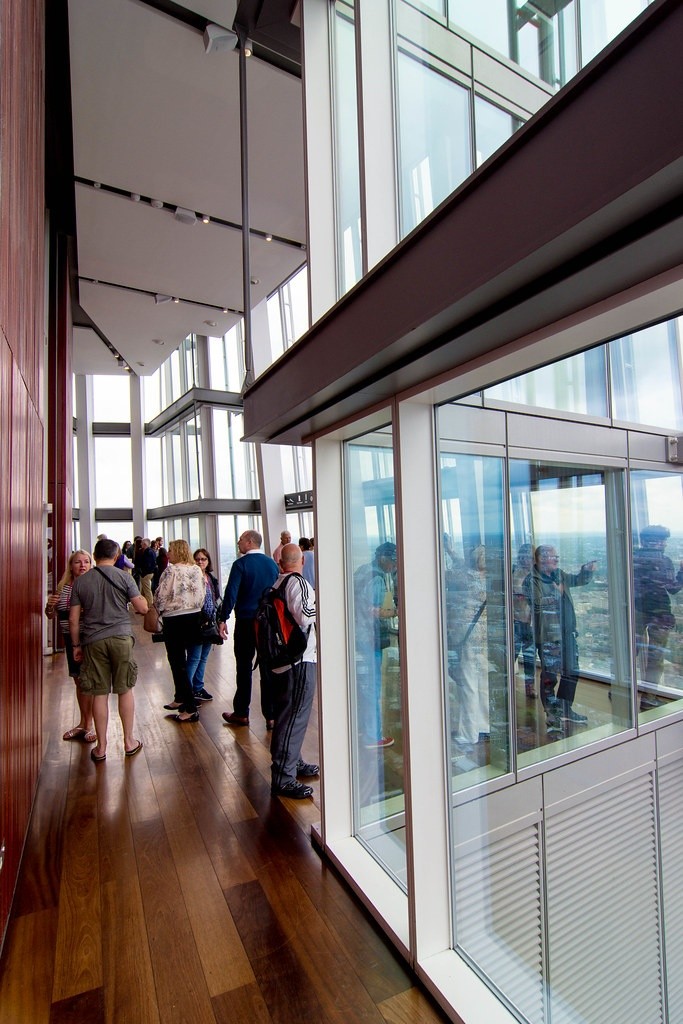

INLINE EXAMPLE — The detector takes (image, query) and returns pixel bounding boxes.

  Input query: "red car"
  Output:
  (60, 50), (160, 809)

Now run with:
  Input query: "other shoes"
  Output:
(173, 711), (200, 722)
(163, 703), (185, 710)
(478, 732), (490, 742)
(525, 683), (538, 698)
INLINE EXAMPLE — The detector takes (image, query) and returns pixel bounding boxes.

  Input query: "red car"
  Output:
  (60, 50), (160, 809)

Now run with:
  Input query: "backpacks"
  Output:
(253, 572), (311, 669)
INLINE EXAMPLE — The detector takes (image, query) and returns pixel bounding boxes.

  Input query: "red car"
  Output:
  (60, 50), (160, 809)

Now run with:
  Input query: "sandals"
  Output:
(62, 727), (83, 739)
(83, 729), (96, 742)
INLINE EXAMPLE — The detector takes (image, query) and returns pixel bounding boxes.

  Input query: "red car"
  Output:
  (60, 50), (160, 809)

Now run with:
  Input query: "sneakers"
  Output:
(193, 688), (213, 700)
(546, 723), (564, 739)
(559, 706), (587, 723)
(271, 780), (313, 798)
(296, 760), (319, 776)
(640, 694), (667, 710)
(192, 699), (202, 708)
(362, 737), (394, 749)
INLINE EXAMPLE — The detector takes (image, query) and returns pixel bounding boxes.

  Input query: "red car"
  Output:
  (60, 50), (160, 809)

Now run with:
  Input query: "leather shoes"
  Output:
(266, 718), (275, 730)
(222, 712), (249, 726)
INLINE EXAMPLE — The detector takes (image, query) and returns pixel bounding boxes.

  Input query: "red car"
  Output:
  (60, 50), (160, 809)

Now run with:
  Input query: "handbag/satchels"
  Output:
(356, 621), (390, 653)
(447, 646), (468, 687)
(209, 605), (224, 645)
(635, 578), (671, 615)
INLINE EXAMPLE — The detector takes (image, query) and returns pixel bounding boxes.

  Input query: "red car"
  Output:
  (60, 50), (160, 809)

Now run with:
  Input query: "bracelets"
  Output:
(72, 642), (80, 648)
(45, 608), (55, 615)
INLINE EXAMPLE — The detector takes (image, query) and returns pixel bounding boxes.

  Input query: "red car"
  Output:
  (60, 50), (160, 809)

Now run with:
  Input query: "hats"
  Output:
(375, 542), (396, 556)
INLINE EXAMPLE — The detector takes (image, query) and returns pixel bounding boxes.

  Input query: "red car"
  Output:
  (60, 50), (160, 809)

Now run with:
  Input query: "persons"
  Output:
(97, 534), (169, 613)
(44, 550), (97, 742)
(69, 540), (149, 762)
(269, 543), (320, 798)
(217, 530), (279, 728)
(155, 540), (206, 724)
(186, 549), (220, 701)
(353, 523), (683, 751)
(274, 531), (315, 590)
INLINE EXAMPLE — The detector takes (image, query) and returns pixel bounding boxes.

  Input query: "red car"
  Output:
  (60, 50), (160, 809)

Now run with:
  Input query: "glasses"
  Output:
(195, 557), (208, 562)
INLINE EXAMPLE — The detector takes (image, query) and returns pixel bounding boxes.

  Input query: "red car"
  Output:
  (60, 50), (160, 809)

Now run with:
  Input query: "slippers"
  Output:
(91, 746), (106, 761)
(125, 739), (142, 755)
